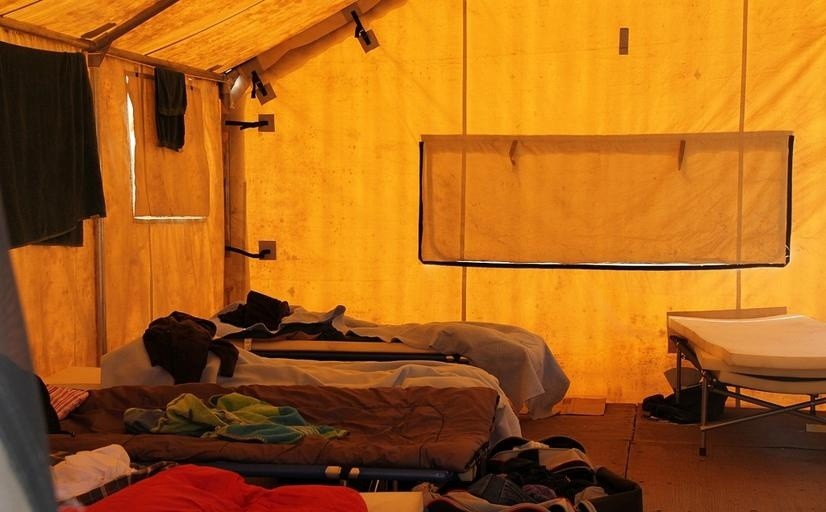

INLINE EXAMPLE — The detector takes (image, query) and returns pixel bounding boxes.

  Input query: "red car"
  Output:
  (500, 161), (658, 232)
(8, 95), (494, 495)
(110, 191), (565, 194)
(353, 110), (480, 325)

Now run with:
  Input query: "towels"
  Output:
(0, 41), (107, 250)
(154, 65), (187, 152)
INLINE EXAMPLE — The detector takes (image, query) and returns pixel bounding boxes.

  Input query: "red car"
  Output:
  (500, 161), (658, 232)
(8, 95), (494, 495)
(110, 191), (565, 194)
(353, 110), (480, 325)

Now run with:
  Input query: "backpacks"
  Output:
(643, 382), (725, 422)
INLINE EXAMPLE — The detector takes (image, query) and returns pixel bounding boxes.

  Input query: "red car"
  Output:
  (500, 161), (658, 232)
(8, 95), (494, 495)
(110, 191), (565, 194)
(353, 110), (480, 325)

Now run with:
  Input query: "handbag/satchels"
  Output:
(428, 435), (643, 511)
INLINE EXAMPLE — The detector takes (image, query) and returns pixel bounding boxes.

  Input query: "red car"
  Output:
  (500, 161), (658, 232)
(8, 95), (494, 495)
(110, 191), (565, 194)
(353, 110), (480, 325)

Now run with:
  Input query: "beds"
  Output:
(48, 421), (569, 512)
(669, 335), (826, 456)
(99, 309), (511, 438)
(205, 290), (569, 415)
